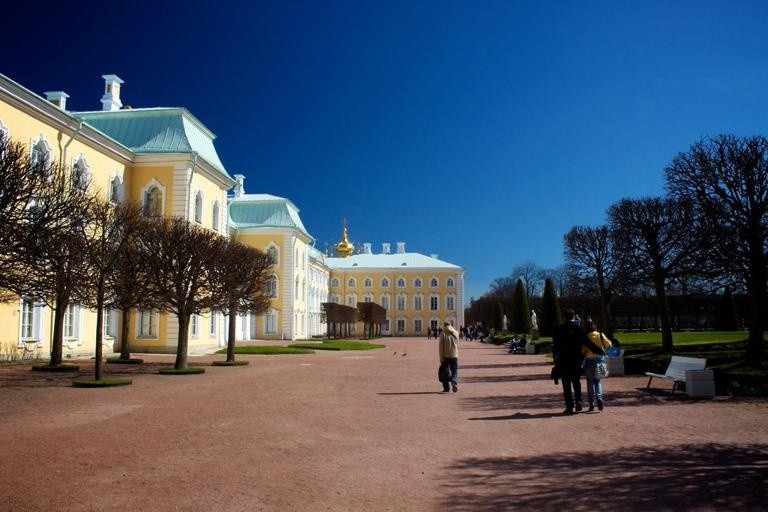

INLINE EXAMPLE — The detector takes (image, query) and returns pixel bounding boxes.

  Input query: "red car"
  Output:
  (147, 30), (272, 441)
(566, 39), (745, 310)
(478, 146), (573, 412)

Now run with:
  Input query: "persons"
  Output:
(552, 308), (605, 416)
(426, 322), (488, 342)
(508, 334), (527, 353)
(529, 308), (539, 330)
(438, 320), (459, 393)
(502, 313), (507, 330)
(581, 320), (611, 412)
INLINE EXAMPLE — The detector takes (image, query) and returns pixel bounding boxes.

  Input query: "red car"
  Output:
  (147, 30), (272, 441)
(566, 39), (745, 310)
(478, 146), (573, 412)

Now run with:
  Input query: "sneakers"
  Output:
(563, 397), (602, 413)
(442, 382), (457, 391)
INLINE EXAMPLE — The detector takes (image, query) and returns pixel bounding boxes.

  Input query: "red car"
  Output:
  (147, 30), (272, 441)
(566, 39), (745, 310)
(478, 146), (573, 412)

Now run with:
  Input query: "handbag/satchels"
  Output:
(593, 361), (610, 378)
(439, 363), (453, 381)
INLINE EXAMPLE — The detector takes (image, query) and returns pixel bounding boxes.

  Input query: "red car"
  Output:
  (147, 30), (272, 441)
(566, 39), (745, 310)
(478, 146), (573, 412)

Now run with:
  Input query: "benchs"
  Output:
(505, 339), (531, 354)
(644, 355), (706, 397)
(604, 347), (625, 376)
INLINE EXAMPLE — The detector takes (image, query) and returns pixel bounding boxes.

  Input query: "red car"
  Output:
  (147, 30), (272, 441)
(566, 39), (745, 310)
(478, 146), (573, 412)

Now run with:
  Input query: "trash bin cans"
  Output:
(526, 344), (535, 354)
(607, 357), (624, 377)
(686, 370), (714, 399)
